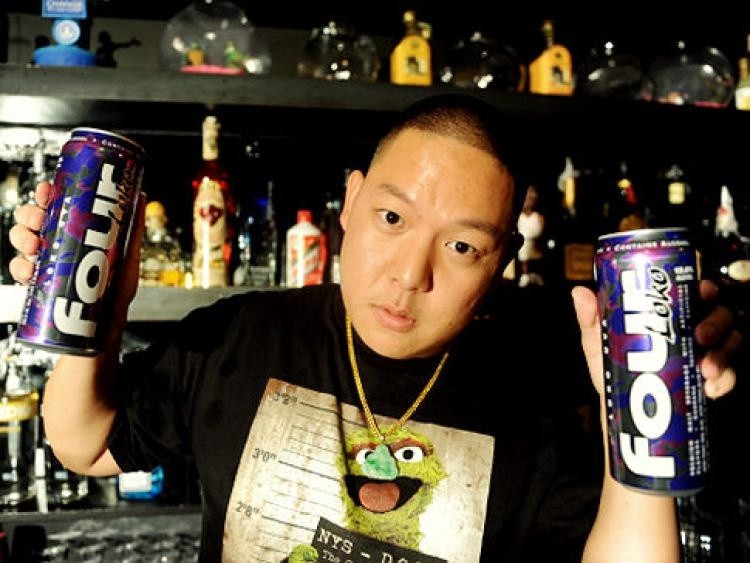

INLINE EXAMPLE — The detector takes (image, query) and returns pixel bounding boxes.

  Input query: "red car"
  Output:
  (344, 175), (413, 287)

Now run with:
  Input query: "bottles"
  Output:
(504, 157), (750, 293)
(1, 323), (163, 514)
(161, 0), (750, 109)
(1, 115), (341, 290)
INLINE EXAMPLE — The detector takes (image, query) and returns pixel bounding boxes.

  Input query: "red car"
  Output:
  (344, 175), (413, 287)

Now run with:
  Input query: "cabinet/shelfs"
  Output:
(0, 61), (750, 548)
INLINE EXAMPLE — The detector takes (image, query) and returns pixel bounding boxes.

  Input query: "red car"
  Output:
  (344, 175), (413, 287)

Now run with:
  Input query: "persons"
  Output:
(9, 94), (744, 563)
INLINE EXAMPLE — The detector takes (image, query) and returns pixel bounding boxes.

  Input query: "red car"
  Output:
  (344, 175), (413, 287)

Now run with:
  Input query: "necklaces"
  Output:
(346, 311), (449, 481)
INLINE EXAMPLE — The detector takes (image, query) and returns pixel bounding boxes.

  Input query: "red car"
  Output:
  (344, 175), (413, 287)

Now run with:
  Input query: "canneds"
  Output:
(594, 226), (713, 496)
(16, 126), (147, 357)
